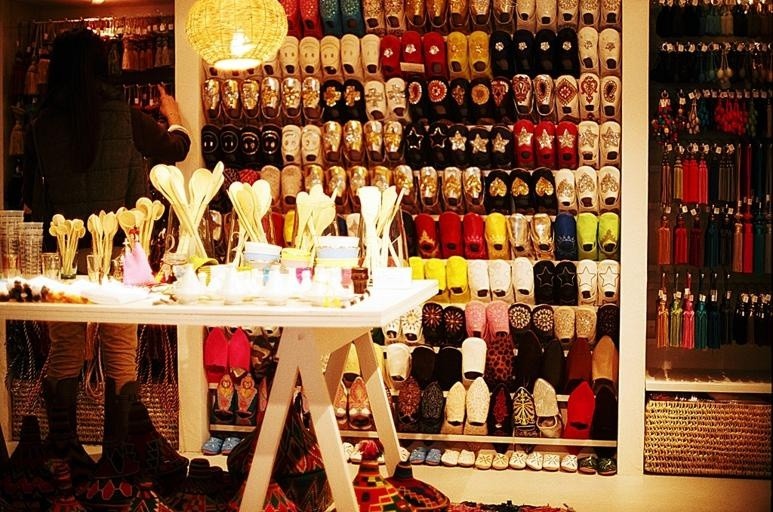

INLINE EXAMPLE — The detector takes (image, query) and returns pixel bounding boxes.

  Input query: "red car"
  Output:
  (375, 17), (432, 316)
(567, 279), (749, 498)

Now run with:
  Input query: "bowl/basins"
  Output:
(315, 236), (359, 283)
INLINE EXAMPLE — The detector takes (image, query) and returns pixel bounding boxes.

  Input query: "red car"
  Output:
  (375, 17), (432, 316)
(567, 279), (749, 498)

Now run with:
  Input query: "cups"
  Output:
(17, 223), (43, 277)
(0, 210), (24, 276)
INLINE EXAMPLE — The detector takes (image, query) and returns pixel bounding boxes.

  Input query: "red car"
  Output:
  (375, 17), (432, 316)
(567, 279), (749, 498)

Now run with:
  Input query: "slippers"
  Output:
(401, 30), (425, 77)
(417, 166), (442, 214)
(509, 168), (536, 214)
(468, 126), (490, 168)
(236, 414), (256, 439)
(426, 0), (448, 36)
(513, 119), (535, 167)
(383, 0), (407, 37)
(213, 375), (236, 414)
(566, 382), (596, 426)
(415, 214), (441, 258)
(591, 335), (617, 395)
(579, 154), (599, 169)
(509, 450), (527, 469)
(342, 120), (365, 167)
(533, 378), (562, 419)
(278, 0), (302, 40)
(343, 78), (364, 122)
(449, 78), (473, 123)
(515, 0), (536, 31)
(579, 102), (600, 124)
(383, 121), (404, 168)
(486, 301), (509, 342)
(462, 166), (486, 214)
(422, 302), (443, 345)
(321, 79), (343, 123)
(348, 422), (372, 444)
(475, 449), (495, 470)
(577, 201), (598, 215)
(301, 125), (322, 165)
(360, 34), (384, 81)
(220, 79), (243, 125)
(426, 76), (451, 120)
(240, 126), (261, 169)
(489, 260), (515, 303)
(469, 59), (492, 81)
(575, 305), (597, 347)
(598, 166), (620, 204)
(302, 77), (324, 127)
(601, 105), (621, 126)
(425, 258), (449, 303)
(511, 74), (535, 122)
(468, 260), (491, 303)
(442, 306), (465, 346)
(461, 336), (487, 390)
(554, 212), (576, 261)
(469, 31), (489, 68)
(556, 121), (577, 169)
(447, 32), (467, 70)
(405, 0), (427, 34)
(575, 166), (597, 205)
(221, 436), (240, 455)
(409, 447), (427, 464)
(492, 452), (508, 470)
(220, 124), (240, 169)
(465, 300), (486, 340)
(279, 36), (300, 79)
(542, 338), (564, 394)
(211, 415), (234, 436)
(419, 383), (445, 447)
(404, 122), (426, 168)
(260, 165), (280, 212)
(400, 447), (410, 462)
(334, 381), (348, 442)
(385, 343), (410, 392)
(601, 76), (621, 115)
(579, 72), (600, 110)
(448, 65), (469, 80)
(514, 330), (541, 393)
(436, 346), (462, 392)
(261, 124), (281, 166)
(490, 30), (512, 80)
(470, 77), (499, 126)
(536, 0), (557, 32)
(362, 0), (385, 35)
(563, 424), (591, 456)
(512, 29), (534, 79)
(492, 0), (515, 31)
(422, 32), (447, 79)
(201, 124), (220, 168)
(441, 421), (463, 449)
(483, 170), (512, 215)
(557, 27), (579, 78)
(531, 305), (554, 342)
(299, 36), (322, 80)
(508, 302), (532, 340)
(401, 306), (423, 346)
(465, 377), (491, 425)
(598, 213), (620, 251)
(526, 451), (543, 470)
(484, 213), (511, 260)
(260, 77), (281, 125)
(558, 0), (579, 31)
(564, 338), (591, 394)
(280, 164), (303, 213)
(380, 35), (403, 79)
(281, 124), (301, 168)
(598, 261), (619, 304)
(425, 448), (441, 465)
(598, 241), (619, 261)
(406, 77), (431, 121)
(533, 74), (557, 123)
(512, 386), (538, 453)
(533, 260), (555, 305)
(599, 196), (621, 214)
(491, 76), (514, 124)
(381, 317), (401, 345)
(325, 166), (351, 214)
(441, 167), (465, 214)
(346, 166), (369, 212)
(299, 0), (323, 40)
(446, 255), (469, 304)
(280, 78), (302, 125)
(385, 77), (411, 124)
(578, 27), (599, 67)
(396, 376), (421, 448)
(411, 346), (436, 390)
(576, 213), (598, 250)
(457, 449), (475, 466)
(561, 454), (578, 471)
(555, 261), (577, 306)
(578, 456), (597, 473)
(598, 458), (616, 475)
(322, 121), (344, 168)
(490, 124), (513, 170)
(554, 306), (575, 349)
(536, 416), (562, 438)
(427, 123), (448, 167)
(234, 373), (258, 415)
(535, 121), (556, 168)
(201, 79), (224, 124)
(262, 61), (281, 78)
(446, 382), (466, 423)
(579, 0), (599, 31)
(530, 214), (555, 260)
(320, 36), (342, 80)
(532, 167), (557, 215)
(488, 383), (512, 453)
(542, 453), (560, 471)
(486, 337), (514, 389)
(448, 0), (470, 34)
(600, 0), (621, 32)
(591, 386), (617, 457)
(507, 213), (535, 260)
(228, 327), (250, 384)
(369, 166), (391, 192)
(220, 169), (240, 198)
(597, 303), (619, 347)
(577, 260), (596, 304)
(463, 212), (487, 260)
(577, 121), (599, 158)
(441, 448), (459, 466)
(600, 57), (621, 78)
(600, 122), (621, 159)
(343, 344), (361, 389)
(468, 0), (491, 33)
(372, 343), (385, 381)
(238, 169), (258, 186)
(463, 421), (488, 452)
(534, 29), (557, 78)
(599, 28), (620, 67)
(201, 436), (222, 455)
(579, 56), (599, 76)
(319, 0), (342, 37)
(364, 80), (386, 122)
(600, 148), (621, 169)
(348, 377), (371, 423)
(557, 203), (577, 214)
(512, 257), (535, 304)
(446, 124), (469, 166)
(555, 75), (579, 123)
(555, 168), (575, 205)
(350, 444), (362, 462)
(304, 163), (324, 192)
(343, 442), (354, 461)
(363, 120), (386, 167)
(438, 211), (464, 257)
(392, 165), (420, 213)
(204, 329), (228, 383)
(340, 0), (364, 38)
(577, 243), (597, 261)
(340, 34), (364, 81)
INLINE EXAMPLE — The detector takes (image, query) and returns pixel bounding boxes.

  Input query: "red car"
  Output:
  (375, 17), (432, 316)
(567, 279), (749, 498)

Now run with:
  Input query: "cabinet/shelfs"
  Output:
(173, 0), (651, 476)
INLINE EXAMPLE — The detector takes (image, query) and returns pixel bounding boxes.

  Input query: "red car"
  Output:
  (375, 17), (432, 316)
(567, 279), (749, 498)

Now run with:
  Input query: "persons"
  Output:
(20, 28), (191, 450)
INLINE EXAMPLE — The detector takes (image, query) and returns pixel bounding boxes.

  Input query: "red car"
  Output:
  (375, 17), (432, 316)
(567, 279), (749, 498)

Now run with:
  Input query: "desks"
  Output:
(0, 274), (440, 512)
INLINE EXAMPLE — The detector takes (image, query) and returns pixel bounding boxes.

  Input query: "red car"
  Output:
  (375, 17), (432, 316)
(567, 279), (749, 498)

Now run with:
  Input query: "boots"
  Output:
(95, 376), (139, 479)
(40, 376), (95, 470)
(238, 79), (261, 125)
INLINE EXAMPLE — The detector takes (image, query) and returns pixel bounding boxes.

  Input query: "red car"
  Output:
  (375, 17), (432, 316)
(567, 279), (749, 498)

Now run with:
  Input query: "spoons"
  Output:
(116, 198), (165, 255)
(294, 183), (336, 251)
(356, 186), (407, 283)
(226, 179), (273, 266)
(150, 161), (225, 258)
(87, 210), (118, 285)
(49, 214), (86, 280)
